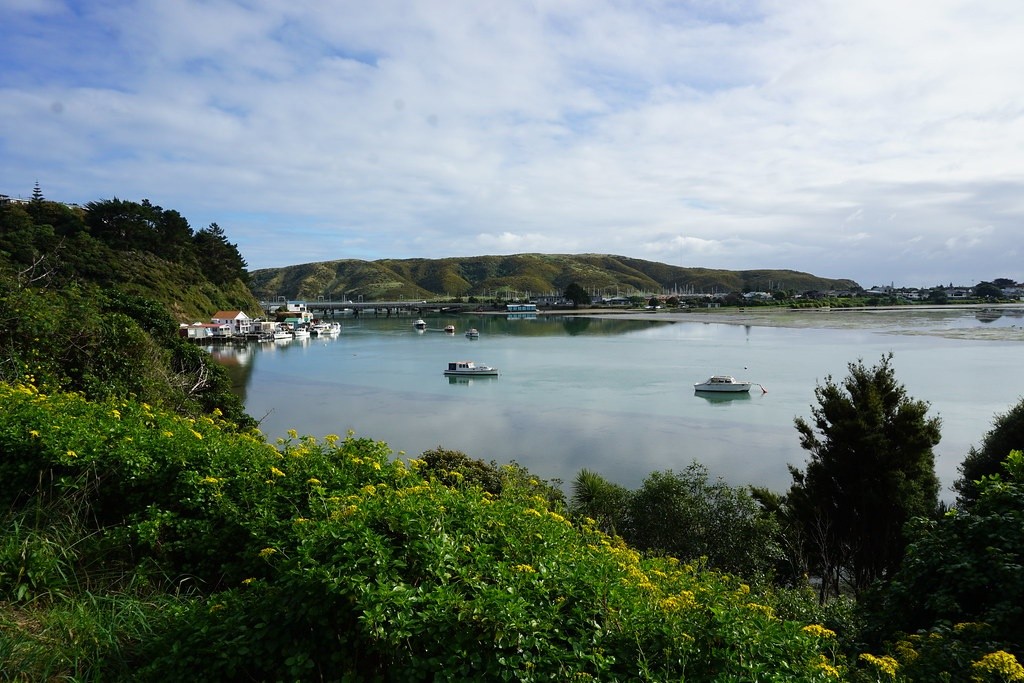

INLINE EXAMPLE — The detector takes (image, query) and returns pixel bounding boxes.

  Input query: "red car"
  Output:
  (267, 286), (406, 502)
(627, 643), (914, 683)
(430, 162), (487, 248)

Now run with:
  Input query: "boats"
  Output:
(444, 362), (499, 376)
(444, 325), (455, 333)
(694, 391), (751, 404)
(466, 327), (480, 336)
(449, 377), (477, 385)
(247, 322), (341, 342)
(694, 376), (752, 391)
(414, 318), (426, 329)
